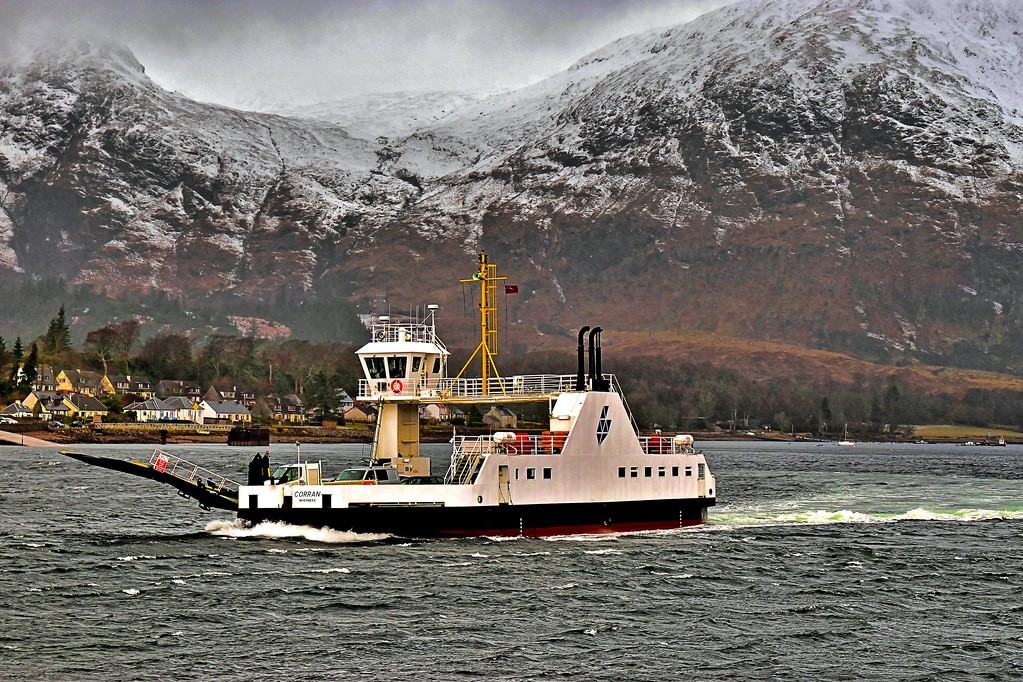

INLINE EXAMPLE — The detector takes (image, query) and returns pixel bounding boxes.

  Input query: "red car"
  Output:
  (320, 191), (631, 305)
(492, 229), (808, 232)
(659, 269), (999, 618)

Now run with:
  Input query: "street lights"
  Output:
(85, 404), (87, 411)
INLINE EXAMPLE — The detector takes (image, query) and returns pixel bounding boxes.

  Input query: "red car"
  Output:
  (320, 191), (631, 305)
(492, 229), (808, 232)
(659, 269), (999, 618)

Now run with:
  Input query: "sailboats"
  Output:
(838, 423), (854, 446)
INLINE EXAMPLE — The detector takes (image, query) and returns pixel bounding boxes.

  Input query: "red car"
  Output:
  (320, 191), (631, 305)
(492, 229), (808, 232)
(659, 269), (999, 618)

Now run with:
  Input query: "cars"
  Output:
(69, 421), (82, 428)
(50, 421), (65, 428)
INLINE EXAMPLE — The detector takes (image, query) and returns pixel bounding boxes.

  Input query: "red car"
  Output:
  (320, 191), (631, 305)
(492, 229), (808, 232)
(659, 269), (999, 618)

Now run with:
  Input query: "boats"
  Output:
(52, 249), (717, 541)
(961, 440), (1006, 447)
(917, 440), (928, 444)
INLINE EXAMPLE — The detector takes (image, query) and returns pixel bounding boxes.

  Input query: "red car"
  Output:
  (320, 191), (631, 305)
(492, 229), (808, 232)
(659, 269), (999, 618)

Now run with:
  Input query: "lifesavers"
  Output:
(391, 380), (403, 393)
